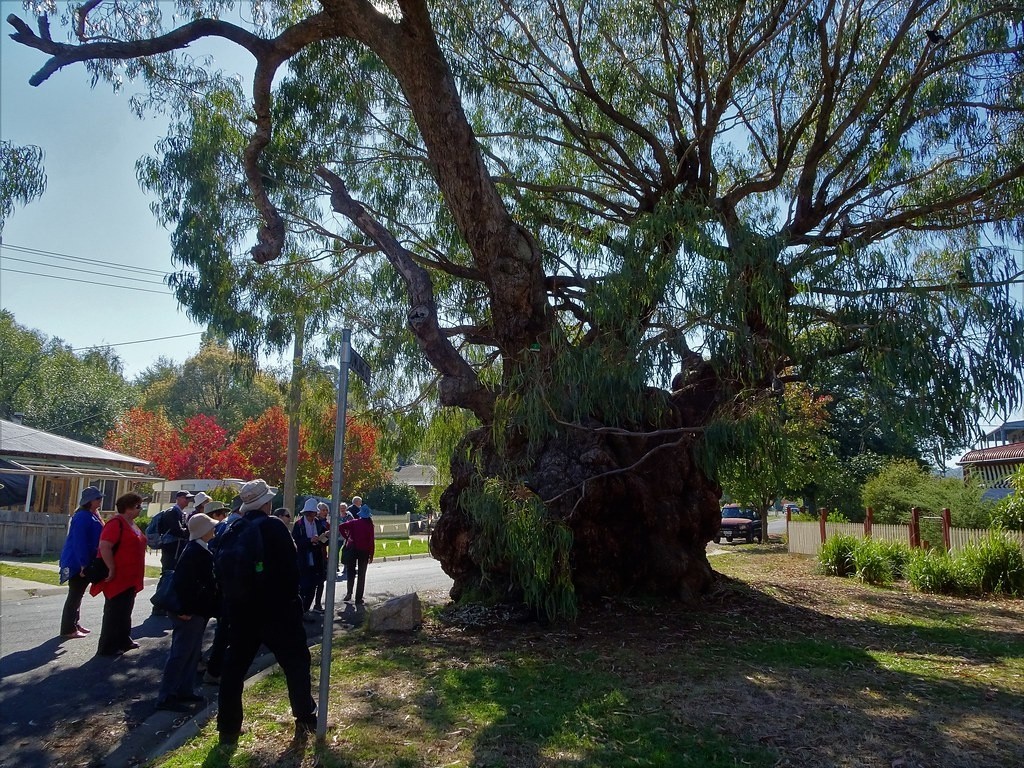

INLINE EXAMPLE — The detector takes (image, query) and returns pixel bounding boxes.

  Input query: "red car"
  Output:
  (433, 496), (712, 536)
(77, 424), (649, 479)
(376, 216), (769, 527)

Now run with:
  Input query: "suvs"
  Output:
(782, 503), (800, 514)
(713, 503), (764, 543)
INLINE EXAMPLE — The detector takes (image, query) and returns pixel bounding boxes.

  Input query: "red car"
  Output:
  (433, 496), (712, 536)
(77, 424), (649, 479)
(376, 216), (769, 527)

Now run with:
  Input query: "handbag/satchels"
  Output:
(342, 532), (365, 564)
(151, 544), (194, 605)
(81, 558), (108, 584)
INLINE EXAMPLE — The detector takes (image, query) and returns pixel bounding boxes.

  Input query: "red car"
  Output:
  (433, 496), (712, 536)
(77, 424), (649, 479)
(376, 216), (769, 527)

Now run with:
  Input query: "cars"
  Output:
(410, 514), (436, 534)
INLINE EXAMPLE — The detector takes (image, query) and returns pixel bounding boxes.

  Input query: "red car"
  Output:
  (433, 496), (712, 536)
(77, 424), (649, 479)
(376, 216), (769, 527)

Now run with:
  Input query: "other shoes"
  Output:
(220, 727), (245, 739)
(344, 593), (351, 600)
(128, 643), (138, 647)
(183, 693), (202, 701)
(305, 612), (312, 616)
(313, 605), (325, 613)
(115, 650), (123, 654)
(156, 703), (189, 711)
(197, 661), (208, 672)
(80, 627), (90, 632)
(355, 600), (364, 604)
(61, 631), (86, 638)
(202, 671), (221, 684)
(296, 719), (335, 737)
(150, 615), (169, 623)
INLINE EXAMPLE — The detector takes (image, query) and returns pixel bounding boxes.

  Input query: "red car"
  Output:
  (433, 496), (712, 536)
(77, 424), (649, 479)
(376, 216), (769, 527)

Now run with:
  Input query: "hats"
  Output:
(81, 486), (106, 504)
(194, 492), (209, 507)
(176, 490), (195, 499)
(356, 504), (371, 518)
(204, 501), (231, 514)
(188, 513), (219, 541)
(239, 479), (278, 512)
(299, 498), (319, 513)
(232, 495), (242, 511)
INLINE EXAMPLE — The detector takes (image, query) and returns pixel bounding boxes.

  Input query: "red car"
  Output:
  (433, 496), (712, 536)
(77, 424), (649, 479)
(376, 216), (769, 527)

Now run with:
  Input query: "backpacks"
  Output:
(145, 506), (184, 559)
(215, 516), (266, 600)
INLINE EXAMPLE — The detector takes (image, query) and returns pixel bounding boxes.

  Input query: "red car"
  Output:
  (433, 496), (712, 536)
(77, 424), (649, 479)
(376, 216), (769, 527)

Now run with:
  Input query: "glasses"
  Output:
(305, 512), (316, 516)
(282, 514), (290, 518)
(96, 497), (102, 500)
(134, 505), (141, 509)
(321, 509), (328, 513)
(214, 511), (227, 518)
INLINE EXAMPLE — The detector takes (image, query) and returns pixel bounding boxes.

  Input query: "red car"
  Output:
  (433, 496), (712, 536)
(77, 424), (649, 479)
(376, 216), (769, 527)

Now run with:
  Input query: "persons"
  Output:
(155, 513), (220, 711)
(186, 491), (213, 520)
(346, 495), (362, 519)
(336, 502), (355, 573)
(292, 497), (330, 624)
(152, 490), (194, 617)
(204, 501), (230, 523)
(339, 504), (375, 606)
(97, 493), (148, 656)
(215, 479), (338, 742)
(313, 501), (331, 614)
(200, 495), (243, 684)
(58, 485), (105, 639)
(272, 508), (291, 526)
